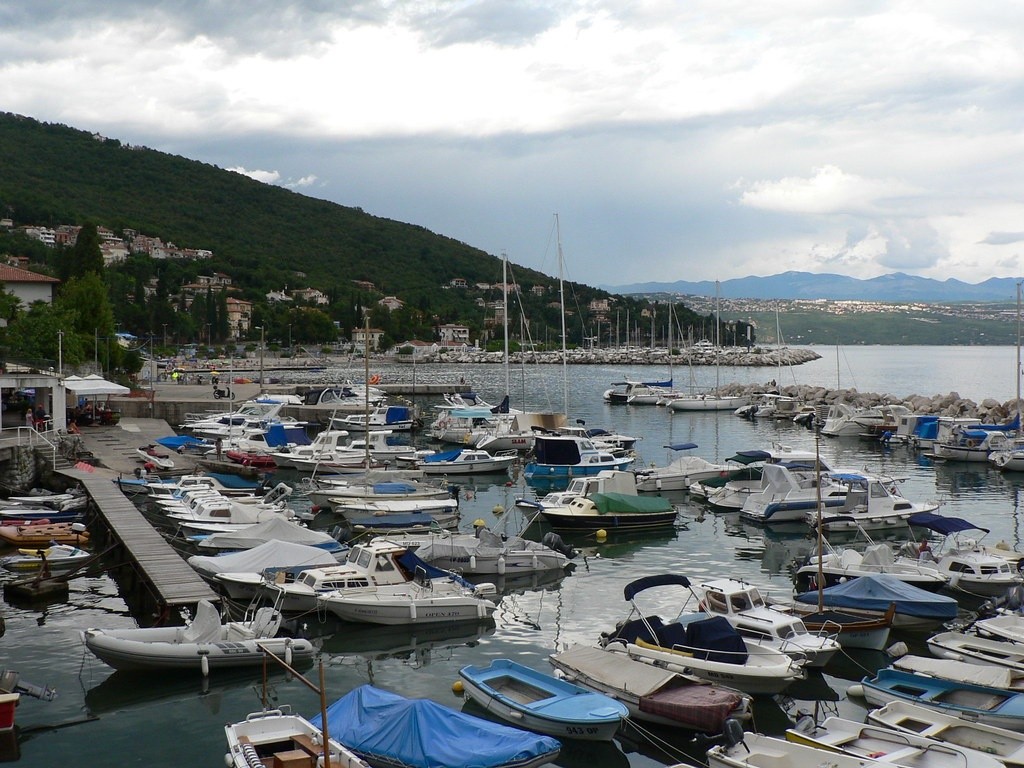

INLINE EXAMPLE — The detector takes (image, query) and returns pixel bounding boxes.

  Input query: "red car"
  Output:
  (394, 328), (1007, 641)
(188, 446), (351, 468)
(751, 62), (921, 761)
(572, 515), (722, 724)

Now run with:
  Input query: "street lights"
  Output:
(289, 324), (292, 346)
(255, 325), (264, 388)
(206, 323), (212, 347)
(162, 324), (168, 348)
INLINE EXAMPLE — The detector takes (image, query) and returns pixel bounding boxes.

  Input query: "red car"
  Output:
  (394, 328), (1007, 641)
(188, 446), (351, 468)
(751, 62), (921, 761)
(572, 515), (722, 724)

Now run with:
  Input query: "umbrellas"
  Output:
(17, 388), (52, 397)
(75, 374), (130, 420)
(61, 374), (81, 386)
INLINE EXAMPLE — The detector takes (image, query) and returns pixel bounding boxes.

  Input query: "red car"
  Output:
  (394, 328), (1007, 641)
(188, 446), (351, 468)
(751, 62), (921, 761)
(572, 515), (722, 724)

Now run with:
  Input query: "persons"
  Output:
(26, 409), (34, 435)
(70, 418), (78, 433)
(73, 397), (111, 424)
(36, 405), (45, 431)
(163, 361), (218, 385)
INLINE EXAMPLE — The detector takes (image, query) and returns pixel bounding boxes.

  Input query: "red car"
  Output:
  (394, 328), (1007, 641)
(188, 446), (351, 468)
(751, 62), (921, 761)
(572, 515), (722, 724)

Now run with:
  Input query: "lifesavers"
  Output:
(370, 375), (377, 383)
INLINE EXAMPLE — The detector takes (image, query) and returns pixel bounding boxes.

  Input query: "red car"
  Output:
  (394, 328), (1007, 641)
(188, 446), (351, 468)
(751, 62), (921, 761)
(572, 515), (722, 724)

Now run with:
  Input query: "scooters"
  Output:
(212, 385), (235, 400)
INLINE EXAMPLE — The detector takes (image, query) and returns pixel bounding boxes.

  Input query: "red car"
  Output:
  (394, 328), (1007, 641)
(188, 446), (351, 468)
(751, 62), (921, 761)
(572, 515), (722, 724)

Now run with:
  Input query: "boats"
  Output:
(78, 662), (315, 720)
(548, 642), (754, 732)
(634, 442), (1024, 631)
(309, 617), (497, 684)
(692, 577), (842, 667)
(0, 484), (91, 604)
(224, 643), (372, 768)
(458, 658), (629, 741)
(696, 418), (896, 651)
(668, 603), (1024, 768)
(309, 684), (561, 768)
(603, 281), (1024, 472)
(110, 209), (678, 624)
(598, 575), (813, 695)
(79, 569), (315, 672)
(0, 670), (58, 733)
